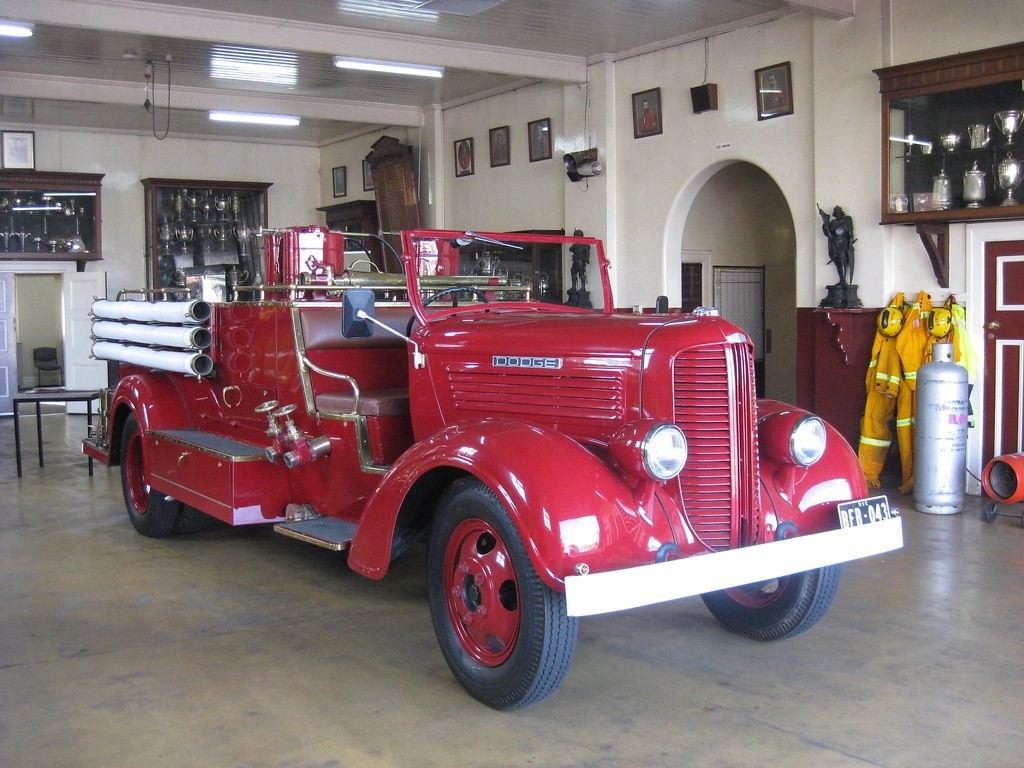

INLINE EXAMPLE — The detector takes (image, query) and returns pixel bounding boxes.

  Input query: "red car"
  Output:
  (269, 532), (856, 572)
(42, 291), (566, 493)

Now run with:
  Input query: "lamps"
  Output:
(563, 151), (605, 182)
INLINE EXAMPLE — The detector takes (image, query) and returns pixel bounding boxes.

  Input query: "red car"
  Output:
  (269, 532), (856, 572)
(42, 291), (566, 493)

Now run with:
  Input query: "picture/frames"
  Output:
(332, 166), (347, 198)
(754, 60), (794, 121)
(454, 137), (475, 177)
(488, 126), (510, 168)
(0, 129), (36, 172)
(528, 117), (552, 162)
(632, 87), (663, 139)
(362, 160), (375, 192)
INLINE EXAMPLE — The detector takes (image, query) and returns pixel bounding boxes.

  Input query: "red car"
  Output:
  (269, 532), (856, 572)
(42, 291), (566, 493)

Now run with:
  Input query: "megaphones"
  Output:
(563, 149), (595, 172)
(577, 160), (603, 175)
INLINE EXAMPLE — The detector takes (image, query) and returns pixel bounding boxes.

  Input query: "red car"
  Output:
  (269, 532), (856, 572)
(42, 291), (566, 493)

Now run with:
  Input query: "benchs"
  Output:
(289, 306), (542, 466)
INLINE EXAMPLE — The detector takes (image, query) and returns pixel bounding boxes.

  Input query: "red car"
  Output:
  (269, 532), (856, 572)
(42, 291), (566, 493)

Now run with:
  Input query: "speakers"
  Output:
(690, 83), (718, 114)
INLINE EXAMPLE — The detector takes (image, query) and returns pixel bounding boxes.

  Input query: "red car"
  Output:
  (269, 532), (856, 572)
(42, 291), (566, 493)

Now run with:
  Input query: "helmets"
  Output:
(877, 307), (903, 337)
(928, 308), (951, 339)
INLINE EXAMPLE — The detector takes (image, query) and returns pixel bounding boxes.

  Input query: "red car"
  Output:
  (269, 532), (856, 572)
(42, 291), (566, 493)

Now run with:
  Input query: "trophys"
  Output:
(462, 249), (550, 301)
(932, 169), (952, 211)
(940, 130), (962, 152)
(968, 121), (992, 149)
(921, 141), (937, 154)
(962, 161), (987, 208)
(994, 109), (1024, 145)
(905, 134), (915, 155)
(157, 189), (258, 301)
(889, 196), (908, 211)
(992, 150), (1024, 207)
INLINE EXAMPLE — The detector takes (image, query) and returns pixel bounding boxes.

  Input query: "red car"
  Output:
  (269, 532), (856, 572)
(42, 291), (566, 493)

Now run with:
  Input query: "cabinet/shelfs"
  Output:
(458, 229), (565, 307)
(316, 199), (385, 274)
(0, 171), (106, 272)
(871, 40), (1024, 289)
(140, 178), (273, 303)
(812, 307), (892, 478)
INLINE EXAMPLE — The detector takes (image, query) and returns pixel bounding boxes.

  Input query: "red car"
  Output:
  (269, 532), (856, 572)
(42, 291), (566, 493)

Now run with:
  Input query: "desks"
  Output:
(13, 390), (100, 478)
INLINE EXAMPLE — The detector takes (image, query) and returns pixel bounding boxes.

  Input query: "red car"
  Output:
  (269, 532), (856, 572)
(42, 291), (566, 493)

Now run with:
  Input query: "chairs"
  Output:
(34, 347), (64, 387)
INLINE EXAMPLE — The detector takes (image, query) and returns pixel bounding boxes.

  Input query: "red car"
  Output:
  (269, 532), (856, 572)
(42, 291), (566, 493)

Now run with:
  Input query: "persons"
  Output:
(10, 138), (27, 163)
(819, 205), (853, 285)
(567, 230), (591, 293)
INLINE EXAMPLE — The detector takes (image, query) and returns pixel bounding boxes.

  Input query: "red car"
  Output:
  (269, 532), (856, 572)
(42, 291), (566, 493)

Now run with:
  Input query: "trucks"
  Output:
(81, 225), (905, 712)
